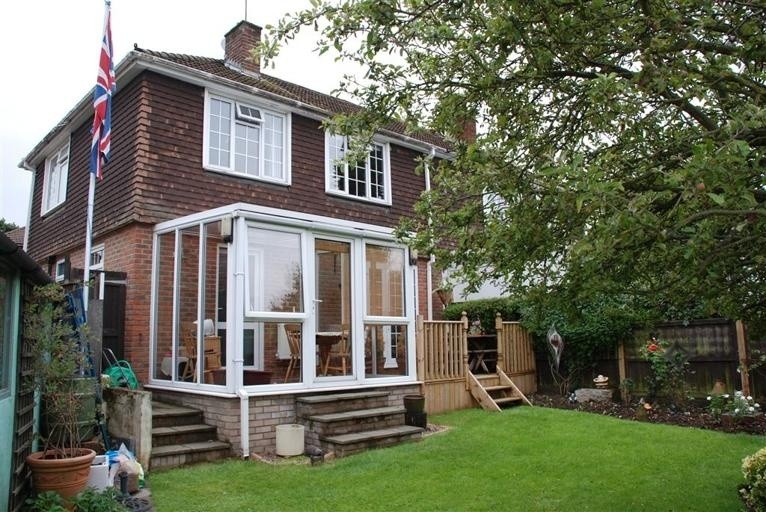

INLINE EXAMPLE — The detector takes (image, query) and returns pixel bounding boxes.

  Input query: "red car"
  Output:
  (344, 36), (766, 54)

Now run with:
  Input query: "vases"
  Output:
(275, 423), (306, 457)
(403, 394), (424, 412)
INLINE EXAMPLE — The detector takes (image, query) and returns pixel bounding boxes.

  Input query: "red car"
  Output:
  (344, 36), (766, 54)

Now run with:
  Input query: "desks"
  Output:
(466, 333), (497, 373)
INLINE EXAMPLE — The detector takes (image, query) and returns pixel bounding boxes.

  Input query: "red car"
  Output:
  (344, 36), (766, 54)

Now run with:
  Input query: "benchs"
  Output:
(466, 348), (497, 373)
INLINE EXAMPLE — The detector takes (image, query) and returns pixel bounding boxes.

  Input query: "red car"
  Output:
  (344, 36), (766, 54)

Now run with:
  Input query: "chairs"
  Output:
(282, 323), (353, 384)
(181, 325), (221, 383)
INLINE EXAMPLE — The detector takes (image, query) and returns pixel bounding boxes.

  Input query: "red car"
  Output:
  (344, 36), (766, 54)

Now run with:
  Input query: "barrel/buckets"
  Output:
(46, 376), (97, 442)
(403, 395), (425, 414)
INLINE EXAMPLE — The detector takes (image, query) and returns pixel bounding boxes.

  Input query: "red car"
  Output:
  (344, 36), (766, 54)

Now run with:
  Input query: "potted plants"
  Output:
(16, 278), (112, 499)
(434, 279), (456, 308)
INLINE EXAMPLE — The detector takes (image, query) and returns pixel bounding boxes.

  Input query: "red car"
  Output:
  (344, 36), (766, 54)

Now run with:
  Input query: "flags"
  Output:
(87, 0), (117, 182)
